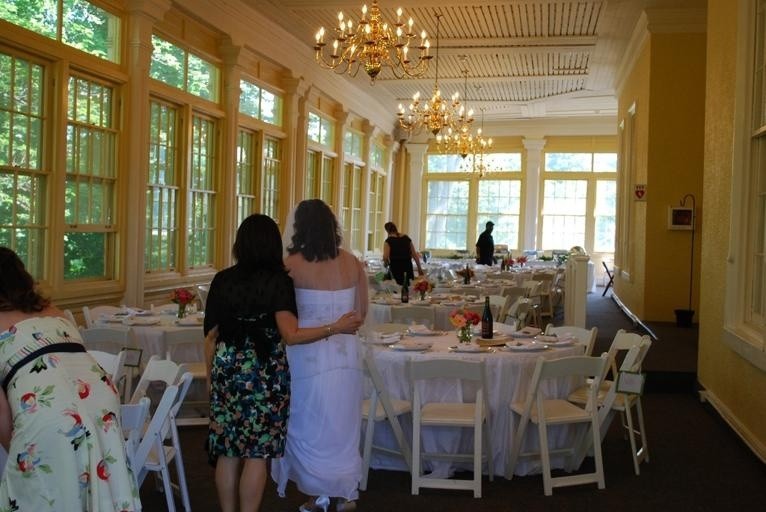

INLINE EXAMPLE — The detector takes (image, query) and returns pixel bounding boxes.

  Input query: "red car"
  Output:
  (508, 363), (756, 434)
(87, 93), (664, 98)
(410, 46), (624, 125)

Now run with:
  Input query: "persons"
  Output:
(475, 221), (497, 266)
(0, 246), (142, 512)
(204, 214), (364, 512)
(271, 199), (368, 512)
(383, 222), (424, 286)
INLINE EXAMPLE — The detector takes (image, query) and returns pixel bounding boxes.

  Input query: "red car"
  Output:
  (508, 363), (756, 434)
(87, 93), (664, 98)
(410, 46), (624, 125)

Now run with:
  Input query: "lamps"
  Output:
(397, 15), (501, 178)
(314, 0), (434, 86)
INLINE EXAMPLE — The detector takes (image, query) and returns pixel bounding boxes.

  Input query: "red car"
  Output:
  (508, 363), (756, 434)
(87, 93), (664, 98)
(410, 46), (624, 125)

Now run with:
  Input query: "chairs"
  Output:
(64, 279), (218, 512)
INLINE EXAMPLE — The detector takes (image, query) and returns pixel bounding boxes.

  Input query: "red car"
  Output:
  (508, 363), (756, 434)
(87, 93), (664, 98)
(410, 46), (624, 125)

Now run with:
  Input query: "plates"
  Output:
(98, 309), (203, 326)
(438, 278), (513, 289)
(359, 326), (578, 353)
(368, 266), (382, 274)
(368, 293), (485, 306)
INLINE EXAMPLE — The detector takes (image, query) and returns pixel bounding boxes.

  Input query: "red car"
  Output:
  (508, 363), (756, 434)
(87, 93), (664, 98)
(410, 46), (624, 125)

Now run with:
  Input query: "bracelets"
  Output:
(326, 324), (334, 337)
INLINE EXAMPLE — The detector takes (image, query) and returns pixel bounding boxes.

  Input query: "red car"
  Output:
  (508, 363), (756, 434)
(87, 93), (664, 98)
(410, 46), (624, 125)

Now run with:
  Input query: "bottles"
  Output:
(464, 263), (470, 284)
(501, 255), (505, 271)
(423, 253), (427, 262)
(401, 272), (409, 303)
(482, 297), (493, 338)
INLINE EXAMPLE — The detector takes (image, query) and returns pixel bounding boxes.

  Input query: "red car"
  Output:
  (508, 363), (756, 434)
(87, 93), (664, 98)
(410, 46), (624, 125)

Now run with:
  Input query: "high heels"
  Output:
(336, 500), (356, 512)
(299, 495), (330, 512)
